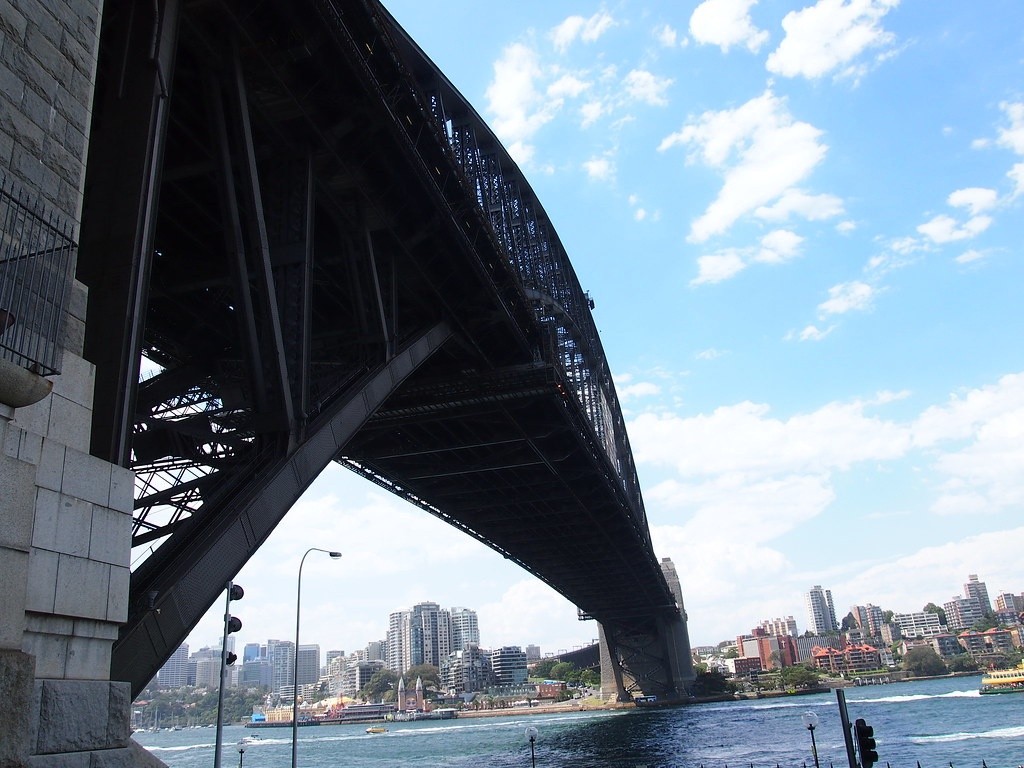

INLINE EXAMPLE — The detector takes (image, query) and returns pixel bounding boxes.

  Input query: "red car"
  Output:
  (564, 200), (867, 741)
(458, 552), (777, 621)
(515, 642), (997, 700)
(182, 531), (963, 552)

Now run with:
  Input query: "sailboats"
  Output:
(148, 706), (161, 733)
(187, 715), (214, 729)
(135, 707), (145, 733)
(169, 711), (182, 733)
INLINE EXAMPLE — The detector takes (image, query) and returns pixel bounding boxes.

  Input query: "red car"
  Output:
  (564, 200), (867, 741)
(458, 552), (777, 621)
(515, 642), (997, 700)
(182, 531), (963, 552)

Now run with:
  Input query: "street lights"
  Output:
(292, 548), (342, 768)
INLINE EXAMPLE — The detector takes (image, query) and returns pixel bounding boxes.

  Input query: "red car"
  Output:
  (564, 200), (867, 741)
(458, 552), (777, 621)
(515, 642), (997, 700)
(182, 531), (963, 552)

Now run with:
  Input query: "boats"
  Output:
(366, 726), (389, 734)
(979, 659), (1024, 695)
(165, 728), (168, 731)
